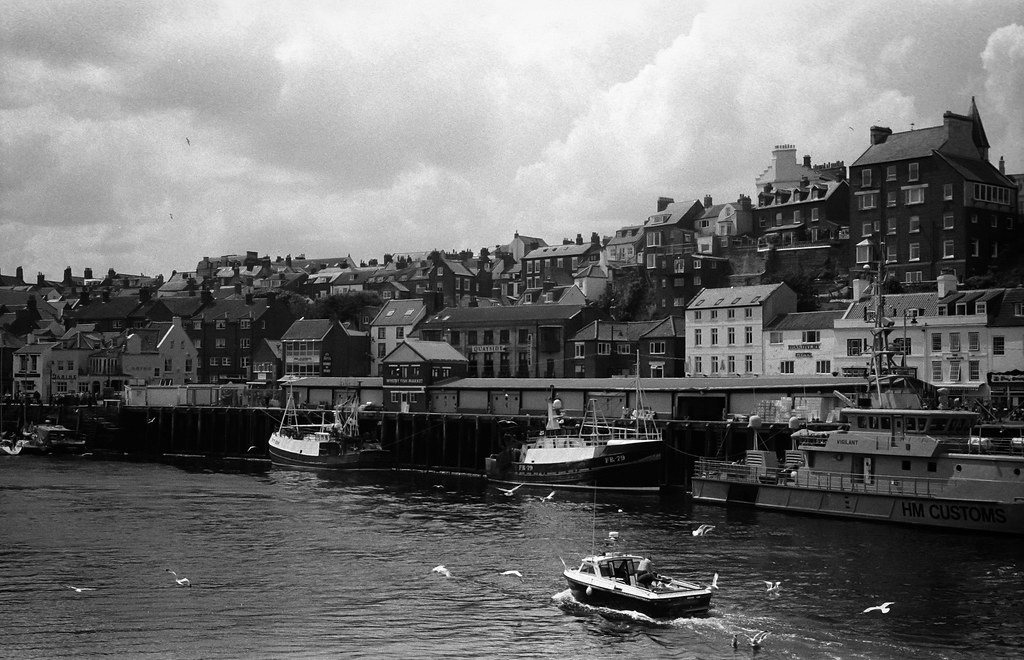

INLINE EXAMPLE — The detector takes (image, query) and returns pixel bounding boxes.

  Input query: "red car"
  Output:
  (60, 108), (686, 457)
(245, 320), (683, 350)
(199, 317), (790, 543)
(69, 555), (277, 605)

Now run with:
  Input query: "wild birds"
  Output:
(499, 568), (522, 578)
(742, 630), (770, 648)
(495, 481), (526, 497)
(691, 523), (716, 537)
(539, 490), (556, 504)
(763, 580), (782, 601)
(710, 572), (720, 590)
(66, 584), (96, 593)
(431, 563), (452, 579)
(863, 601), (895, 614)
(165, 568), (193, 588)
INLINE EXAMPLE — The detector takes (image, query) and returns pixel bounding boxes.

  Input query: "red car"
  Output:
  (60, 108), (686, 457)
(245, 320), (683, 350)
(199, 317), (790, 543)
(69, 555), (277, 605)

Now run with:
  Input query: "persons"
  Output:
(9, 432), (17, 447)
(636, 572), (657, 589)
(636, 556), (653, 579)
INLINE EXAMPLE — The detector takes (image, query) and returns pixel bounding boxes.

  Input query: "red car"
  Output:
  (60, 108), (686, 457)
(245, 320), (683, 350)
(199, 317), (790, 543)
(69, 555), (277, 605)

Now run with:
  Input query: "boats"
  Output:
(563, 483), (715, 621)
(691, 262), (1024, 533)
(485, 349), (665, 490)
(267, 382), (361, 467)
